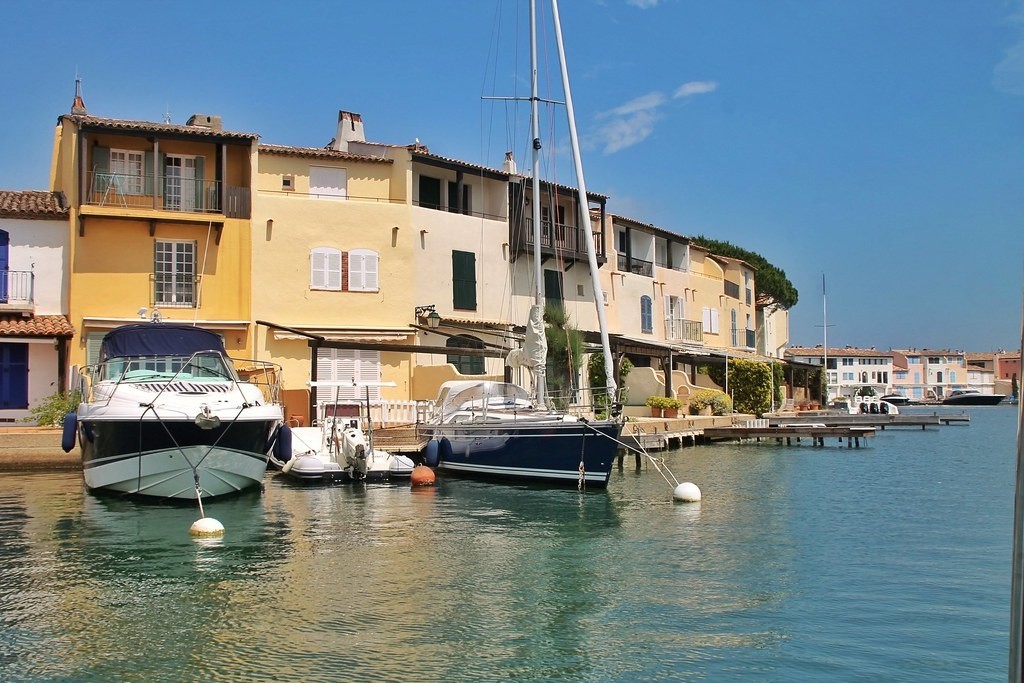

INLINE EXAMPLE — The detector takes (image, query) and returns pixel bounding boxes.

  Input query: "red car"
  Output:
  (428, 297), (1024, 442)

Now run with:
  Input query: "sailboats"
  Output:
(412, 1), (705, 505)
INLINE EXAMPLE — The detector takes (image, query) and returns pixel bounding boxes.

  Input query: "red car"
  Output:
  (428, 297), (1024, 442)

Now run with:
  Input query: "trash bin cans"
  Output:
(859, 403), (868, 413)
(787, 399), (794, 411)
(870, 403), (878, 413)
(1010, 396), (1014, 400)
(881, 402), (888, 414)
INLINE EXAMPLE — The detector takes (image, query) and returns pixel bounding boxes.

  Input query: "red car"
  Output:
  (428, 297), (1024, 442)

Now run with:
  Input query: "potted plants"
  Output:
(647, 395), (681, 417)
(799, 400), (819, 410)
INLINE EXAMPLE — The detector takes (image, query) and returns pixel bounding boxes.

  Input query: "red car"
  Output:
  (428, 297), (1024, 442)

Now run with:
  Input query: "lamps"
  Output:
(415, 305), (441, 329)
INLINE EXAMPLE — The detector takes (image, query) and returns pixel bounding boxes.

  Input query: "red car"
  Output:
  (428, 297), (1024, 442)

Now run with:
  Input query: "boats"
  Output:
(880, 392), (910, 407)
(943, 389), (1007, 406)
(269, 375), (436, 487)
(61, 218), (286, 537)
